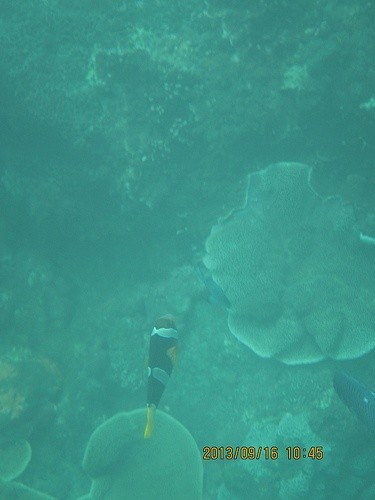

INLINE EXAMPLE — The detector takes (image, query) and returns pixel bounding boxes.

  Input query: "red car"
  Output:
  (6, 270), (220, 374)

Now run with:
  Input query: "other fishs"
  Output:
(142, 314), (179, 440)
